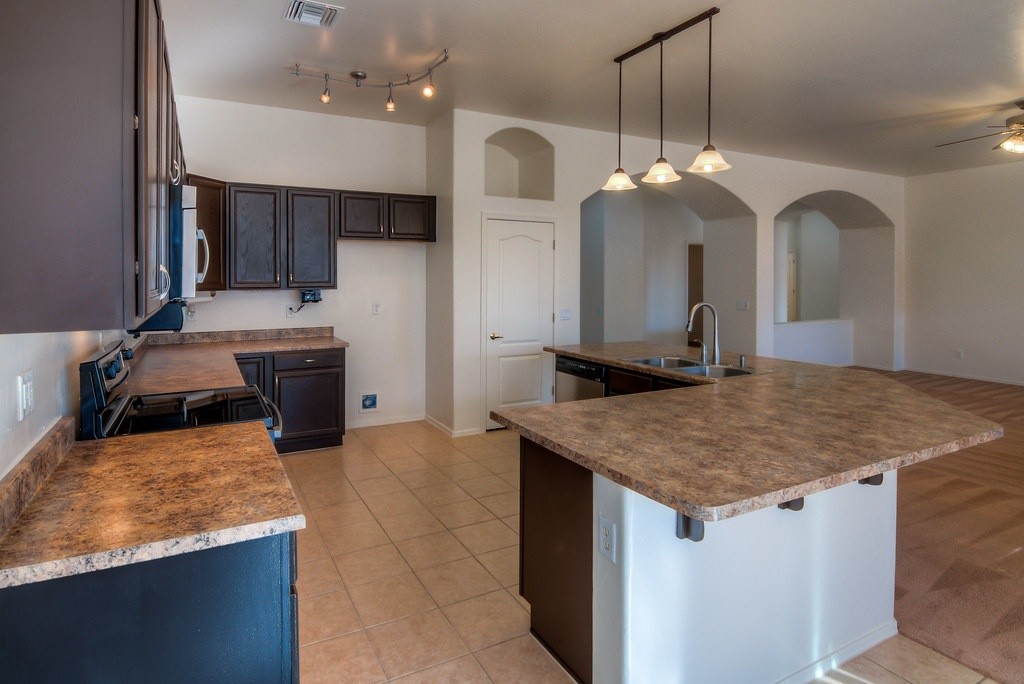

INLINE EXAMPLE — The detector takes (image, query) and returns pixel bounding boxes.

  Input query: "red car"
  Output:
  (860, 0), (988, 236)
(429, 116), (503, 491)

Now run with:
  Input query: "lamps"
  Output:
(601, 7), (733, 192)
(295, 47), (450, 111)
(993, 125), (1024, 155)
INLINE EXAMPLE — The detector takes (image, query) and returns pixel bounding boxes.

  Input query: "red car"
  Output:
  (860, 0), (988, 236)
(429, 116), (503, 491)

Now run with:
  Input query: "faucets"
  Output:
(685, 302), (721, 365)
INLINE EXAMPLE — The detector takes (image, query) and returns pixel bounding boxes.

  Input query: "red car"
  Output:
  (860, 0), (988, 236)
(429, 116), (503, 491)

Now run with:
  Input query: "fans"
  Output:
(934, 100), (1024, 150)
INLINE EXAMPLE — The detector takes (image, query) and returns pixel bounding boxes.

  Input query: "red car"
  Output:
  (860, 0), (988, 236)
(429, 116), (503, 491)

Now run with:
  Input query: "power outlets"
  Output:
(597, 512), (617, 562)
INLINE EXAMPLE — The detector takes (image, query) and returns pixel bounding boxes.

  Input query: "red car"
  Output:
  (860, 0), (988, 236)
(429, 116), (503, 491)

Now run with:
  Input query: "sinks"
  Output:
(672, 368), (752, 377)
(617, 356), (700, 368)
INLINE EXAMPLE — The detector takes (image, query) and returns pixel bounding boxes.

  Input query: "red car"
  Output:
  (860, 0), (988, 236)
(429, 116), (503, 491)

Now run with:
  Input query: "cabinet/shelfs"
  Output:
(604, 369), (683, 397)
(0, 0), (436, 334)
(222, 347), (349, 454)
(518, 433), (593, 684)
(1, 530), (300, 684)
